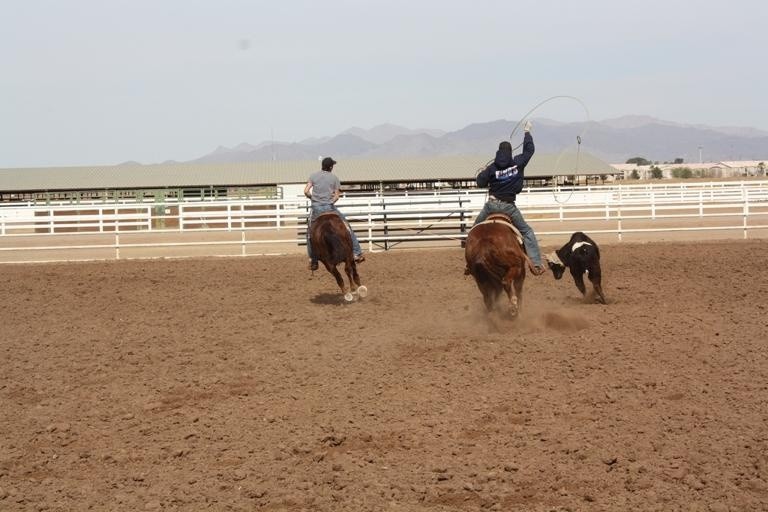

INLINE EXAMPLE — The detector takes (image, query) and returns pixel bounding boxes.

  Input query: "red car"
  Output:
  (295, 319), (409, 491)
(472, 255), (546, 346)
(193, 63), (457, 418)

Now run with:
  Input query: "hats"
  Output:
(495, 142), (511, 169)
(322, 158), (336, 168)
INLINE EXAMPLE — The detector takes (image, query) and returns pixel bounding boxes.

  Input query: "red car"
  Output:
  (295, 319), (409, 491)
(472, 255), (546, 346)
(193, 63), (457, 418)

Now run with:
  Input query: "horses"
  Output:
(309, 212), (369, 302)
(464, 213), (526, 321)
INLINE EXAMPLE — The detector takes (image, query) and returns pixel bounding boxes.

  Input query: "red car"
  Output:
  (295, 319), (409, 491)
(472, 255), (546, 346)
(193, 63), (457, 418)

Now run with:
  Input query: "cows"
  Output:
(542, 231), (607, 304)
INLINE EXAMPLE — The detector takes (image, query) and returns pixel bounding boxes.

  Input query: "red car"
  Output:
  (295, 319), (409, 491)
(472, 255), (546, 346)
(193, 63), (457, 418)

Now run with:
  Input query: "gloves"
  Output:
(524, 120), (532, 132)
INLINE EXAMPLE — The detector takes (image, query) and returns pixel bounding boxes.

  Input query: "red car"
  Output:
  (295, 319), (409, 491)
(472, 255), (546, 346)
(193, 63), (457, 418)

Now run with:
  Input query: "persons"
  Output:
(462, 122), (548, 279)
(304, 157), (365, 269)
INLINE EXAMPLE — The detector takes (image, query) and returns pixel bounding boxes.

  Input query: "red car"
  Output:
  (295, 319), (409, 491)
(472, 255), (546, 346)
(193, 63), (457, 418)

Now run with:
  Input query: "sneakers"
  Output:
(465, 265), (471, 276)
(533, 266), (542, 272)
(312, 265), (318, 269)
(354, 255), (365, 262)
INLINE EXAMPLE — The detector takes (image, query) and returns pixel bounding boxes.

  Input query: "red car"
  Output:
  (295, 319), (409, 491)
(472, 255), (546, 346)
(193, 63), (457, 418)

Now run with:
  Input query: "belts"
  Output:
(489, 198), (505, 202)
